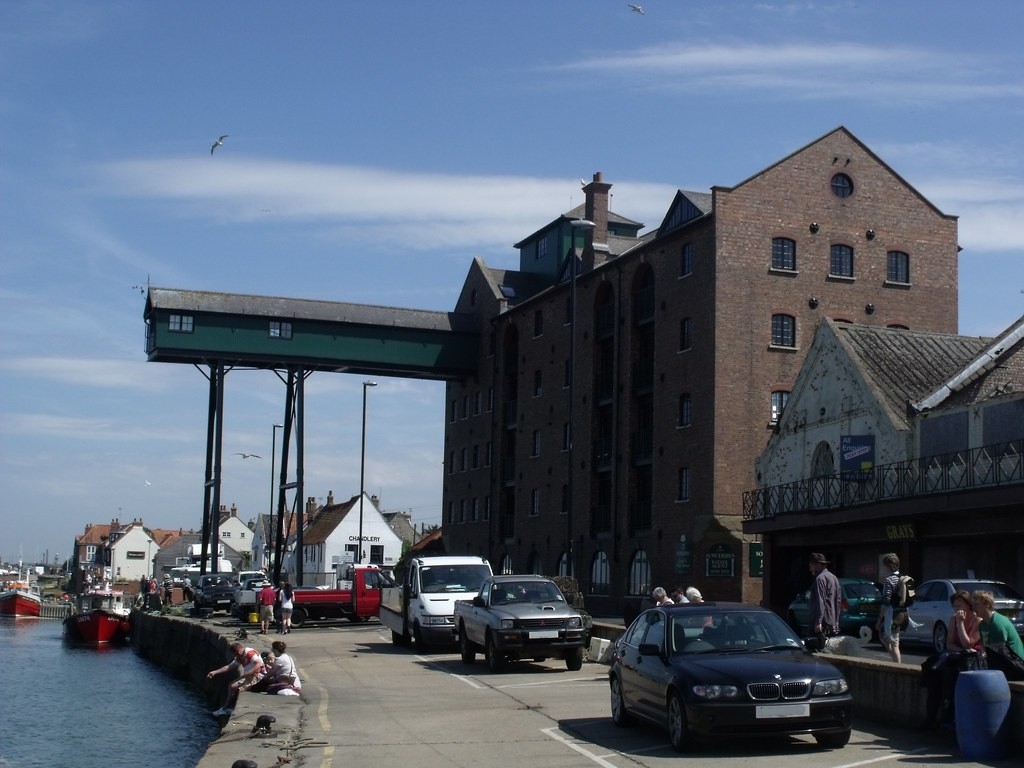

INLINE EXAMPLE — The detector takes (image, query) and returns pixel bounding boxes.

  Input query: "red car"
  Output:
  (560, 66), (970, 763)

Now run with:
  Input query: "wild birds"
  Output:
(210, 135), (230, 155)
(145, 480), (151, 486)
(232, 453), (261, 459)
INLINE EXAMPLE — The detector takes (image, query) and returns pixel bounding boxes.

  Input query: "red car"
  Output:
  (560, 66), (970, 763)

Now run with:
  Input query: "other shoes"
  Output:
(260, 629), (265, 634)
(212, 707), (232, 716)
(287, 627), (291, 634)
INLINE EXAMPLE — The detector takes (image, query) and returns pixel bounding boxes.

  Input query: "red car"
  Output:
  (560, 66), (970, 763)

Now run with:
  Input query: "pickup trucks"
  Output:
(274, 564), (400, 628)
(454, 574), (589, 672)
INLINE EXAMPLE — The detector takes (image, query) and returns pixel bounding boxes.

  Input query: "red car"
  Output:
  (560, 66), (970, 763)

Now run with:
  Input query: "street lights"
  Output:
(268, 422), (283, 578)
(357, 380), (377, 565)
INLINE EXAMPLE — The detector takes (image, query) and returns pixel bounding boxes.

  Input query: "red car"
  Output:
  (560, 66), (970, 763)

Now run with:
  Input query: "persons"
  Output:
(207, 641), (307, 716)
(652, 587), (713, 629)
(920, 590), (1024, 732)
(140, 574), (192, 606)
(257, 579), (295, 635)
(876, 553), (901, 663)
(808, 553), (841, 652)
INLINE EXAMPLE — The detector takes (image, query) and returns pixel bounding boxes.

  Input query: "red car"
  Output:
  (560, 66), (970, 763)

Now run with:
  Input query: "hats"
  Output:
(808, 552), (829, 564)
(164, 574), (170, 578)
(262, 580), (272, 586)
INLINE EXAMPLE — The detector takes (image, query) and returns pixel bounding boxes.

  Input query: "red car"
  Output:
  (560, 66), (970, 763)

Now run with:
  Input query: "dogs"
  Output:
(824, 626), (872, 658)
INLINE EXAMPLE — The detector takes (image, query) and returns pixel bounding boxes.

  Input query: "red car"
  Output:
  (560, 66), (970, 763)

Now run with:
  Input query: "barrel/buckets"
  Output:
(954, 670), (1011, 759)
(248, 612), (259, 622)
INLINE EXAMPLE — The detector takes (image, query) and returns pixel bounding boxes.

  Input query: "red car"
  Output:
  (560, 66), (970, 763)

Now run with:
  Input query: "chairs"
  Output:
(666, 622), (686, 652)
(491, 589), (505, 604)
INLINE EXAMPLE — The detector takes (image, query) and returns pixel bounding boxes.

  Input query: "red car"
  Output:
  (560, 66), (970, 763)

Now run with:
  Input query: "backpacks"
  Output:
(889, 574), (916, 607)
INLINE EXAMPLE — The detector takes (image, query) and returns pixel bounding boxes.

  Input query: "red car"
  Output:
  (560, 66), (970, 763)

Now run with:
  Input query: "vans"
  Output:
(391, 557), (496, 653)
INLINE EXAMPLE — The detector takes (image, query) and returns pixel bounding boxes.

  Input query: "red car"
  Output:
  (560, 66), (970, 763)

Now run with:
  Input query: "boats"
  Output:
(0, 559), (42, 619)
(61, 568), (135, 649)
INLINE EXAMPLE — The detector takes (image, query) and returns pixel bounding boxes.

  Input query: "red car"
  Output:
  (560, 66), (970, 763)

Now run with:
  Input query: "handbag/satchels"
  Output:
(988, 642), (1024, 681)
(278, 675), (296, 685)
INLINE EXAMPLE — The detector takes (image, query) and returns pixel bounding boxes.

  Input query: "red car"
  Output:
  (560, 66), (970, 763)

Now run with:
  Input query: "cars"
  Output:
(233, 571), (328, 625)
(193, 575), (237, 614)
(897, 577), (1024, 656)
(787, 578), (884, 640)
(607, 601), (855, 753)
